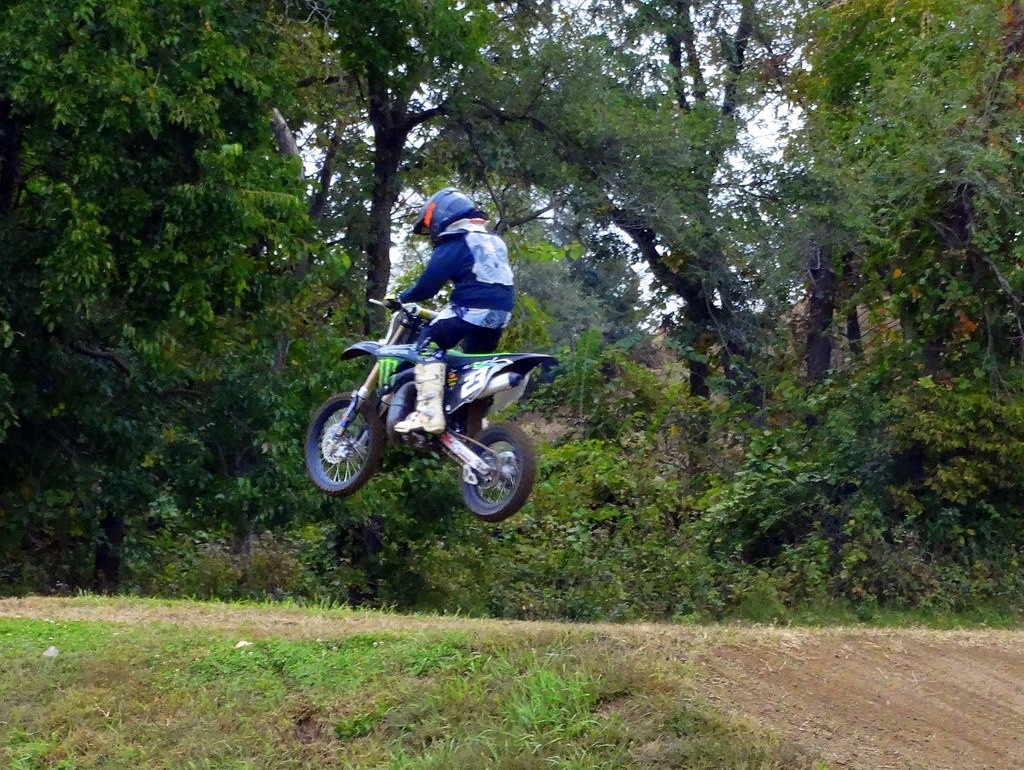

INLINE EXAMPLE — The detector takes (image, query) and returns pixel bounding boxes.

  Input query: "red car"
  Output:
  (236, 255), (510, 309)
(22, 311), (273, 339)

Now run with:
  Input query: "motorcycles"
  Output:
(304, 293), (560, 523)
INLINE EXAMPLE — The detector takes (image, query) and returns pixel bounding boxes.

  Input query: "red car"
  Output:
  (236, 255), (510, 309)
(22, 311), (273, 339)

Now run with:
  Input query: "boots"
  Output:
(393, 363), (446, 436)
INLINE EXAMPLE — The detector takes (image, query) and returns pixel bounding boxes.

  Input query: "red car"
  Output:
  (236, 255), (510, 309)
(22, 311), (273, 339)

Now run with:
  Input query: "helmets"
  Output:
(413, 187), (488, 236)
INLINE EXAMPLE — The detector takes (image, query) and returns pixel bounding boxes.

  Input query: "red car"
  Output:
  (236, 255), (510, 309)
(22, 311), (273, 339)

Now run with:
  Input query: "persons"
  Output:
(384, 185), (516, 434)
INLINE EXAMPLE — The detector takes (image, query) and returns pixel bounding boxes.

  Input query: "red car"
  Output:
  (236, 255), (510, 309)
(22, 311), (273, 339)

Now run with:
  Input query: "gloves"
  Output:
(384, 294), (403, 315)
